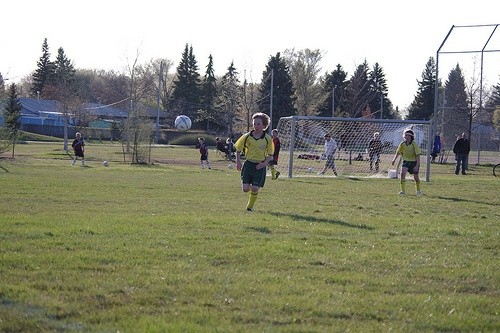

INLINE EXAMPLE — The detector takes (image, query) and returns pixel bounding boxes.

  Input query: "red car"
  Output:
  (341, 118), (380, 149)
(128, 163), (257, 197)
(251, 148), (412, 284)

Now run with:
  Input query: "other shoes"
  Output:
(318, 171), (325, 175)
(398, 191), (405, 195)
(416, 191), (423, 197)
(275, 172), (280, 179)
(246, 207), (254, 212)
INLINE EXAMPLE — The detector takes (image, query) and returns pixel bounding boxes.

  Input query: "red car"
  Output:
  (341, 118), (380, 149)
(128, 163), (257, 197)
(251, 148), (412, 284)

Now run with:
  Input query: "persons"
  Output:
(198, 138), (211, 169)
(318, 134), (338, 175)
(453, 132), (470, 175)
(233, 113), (274, 211)
(392, 130), (422, 195)
(368, 132), (382, 173)
(72, 133), (85, 165)
(216, 138), (229, 155)
(227, 138), (236, 152)
(268, 128), (280, 180)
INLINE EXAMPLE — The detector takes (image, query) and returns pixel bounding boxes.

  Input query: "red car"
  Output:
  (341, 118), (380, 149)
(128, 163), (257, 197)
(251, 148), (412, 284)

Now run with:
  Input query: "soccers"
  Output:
(308, 168), (313, 173)
(228, 164), (233, 169)
(388, 170), (397, 178)
(174, 115), (192, 131)
(103, 161), (109, 167)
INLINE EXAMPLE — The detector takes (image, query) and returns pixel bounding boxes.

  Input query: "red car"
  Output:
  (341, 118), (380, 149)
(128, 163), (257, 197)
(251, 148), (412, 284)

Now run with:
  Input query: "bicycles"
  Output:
(438, 146), (447, 164)
